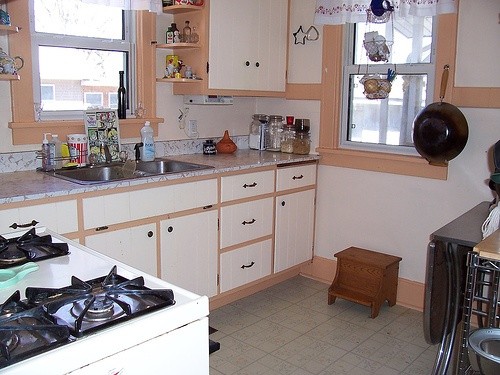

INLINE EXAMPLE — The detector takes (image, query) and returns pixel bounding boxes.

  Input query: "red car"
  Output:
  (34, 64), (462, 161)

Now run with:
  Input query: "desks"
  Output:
(456, 229), (500, 375)
(424, 201), (493, 345)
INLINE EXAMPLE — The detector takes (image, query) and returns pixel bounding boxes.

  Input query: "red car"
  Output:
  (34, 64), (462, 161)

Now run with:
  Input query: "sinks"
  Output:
(44, 167), (148, 185)
(125, 159), (216, 174)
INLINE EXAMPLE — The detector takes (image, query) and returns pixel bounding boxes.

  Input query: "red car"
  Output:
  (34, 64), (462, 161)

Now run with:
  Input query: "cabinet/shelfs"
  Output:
(0, 25), (21, 81)
(0, 200), (80, 237)
(156, 5), (204, 83)
(207, 0), (290, 91)
(272, 163), (317, 275)
(219, 169), (276, 293)
(83, 178), (220, 299)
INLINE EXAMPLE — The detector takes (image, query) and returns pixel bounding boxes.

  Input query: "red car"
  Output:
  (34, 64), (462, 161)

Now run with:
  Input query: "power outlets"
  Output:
(190, 120), (198, 132)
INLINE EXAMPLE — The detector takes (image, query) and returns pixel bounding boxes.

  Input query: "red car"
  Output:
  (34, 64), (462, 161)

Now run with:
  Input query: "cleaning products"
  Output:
(139, 120), (155, 161)
(39, 132), (52, 172)
(50, 134), (63, 169)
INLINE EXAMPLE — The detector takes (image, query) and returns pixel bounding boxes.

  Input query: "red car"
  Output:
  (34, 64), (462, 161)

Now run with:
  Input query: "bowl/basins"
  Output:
(469, 328), (500, 375)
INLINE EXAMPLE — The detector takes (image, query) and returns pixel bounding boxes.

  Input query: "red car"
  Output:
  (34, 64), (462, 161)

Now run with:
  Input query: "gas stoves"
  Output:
(0, 226), (209, 375)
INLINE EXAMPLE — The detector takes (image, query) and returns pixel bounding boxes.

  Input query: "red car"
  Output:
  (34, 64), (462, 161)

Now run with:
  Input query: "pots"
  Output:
(412, 65), (469, 167)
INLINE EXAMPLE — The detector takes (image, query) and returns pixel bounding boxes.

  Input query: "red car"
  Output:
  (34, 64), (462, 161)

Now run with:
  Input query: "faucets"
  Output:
(133, 141), (144, 161)
(86, 144), (128, 168)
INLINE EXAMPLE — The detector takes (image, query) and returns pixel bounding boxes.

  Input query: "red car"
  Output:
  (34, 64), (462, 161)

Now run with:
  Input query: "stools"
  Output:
(327, 246), (401, 318)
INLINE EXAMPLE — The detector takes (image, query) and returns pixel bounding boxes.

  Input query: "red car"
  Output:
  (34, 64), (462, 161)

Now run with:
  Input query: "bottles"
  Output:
(166, 23), (179, 44)
(203, 140), (216, 155)
(162, 0), (173, 7)
(185, 66), (192, 79)
(248, 114), (311, 155)
(118, 70), (126, 118)
(0, 8), (11, 26)
(141, 120), (155, 162)
(183, 21), (191, 43)
(216, 130), (237, 154)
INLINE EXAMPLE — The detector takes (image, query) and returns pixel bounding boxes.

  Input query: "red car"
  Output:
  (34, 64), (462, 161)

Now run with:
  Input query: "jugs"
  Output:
(0, 48), (24, 76)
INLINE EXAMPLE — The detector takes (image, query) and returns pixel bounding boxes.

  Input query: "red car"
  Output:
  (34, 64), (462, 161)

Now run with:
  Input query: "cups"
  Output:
(66, 134), (88, 168)
